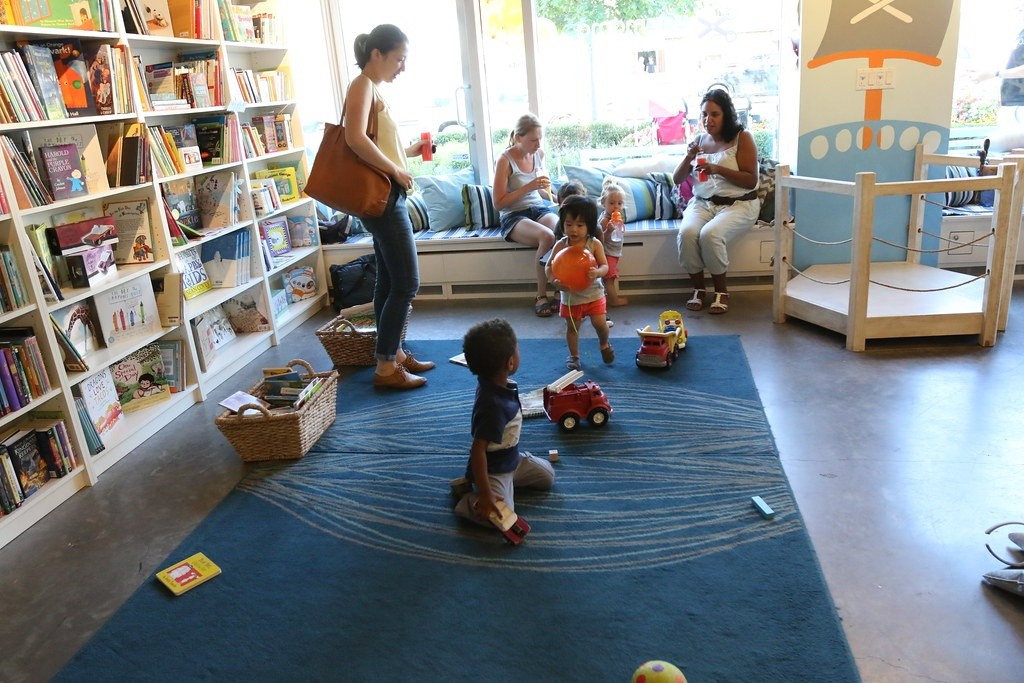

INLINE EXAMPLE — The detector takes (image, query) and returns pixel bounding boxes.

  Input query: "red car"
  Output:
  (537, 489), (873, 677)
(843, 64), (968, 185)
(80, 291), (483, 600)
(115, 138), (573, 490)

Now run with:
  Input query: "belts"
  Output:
(708, 191), (757, 205)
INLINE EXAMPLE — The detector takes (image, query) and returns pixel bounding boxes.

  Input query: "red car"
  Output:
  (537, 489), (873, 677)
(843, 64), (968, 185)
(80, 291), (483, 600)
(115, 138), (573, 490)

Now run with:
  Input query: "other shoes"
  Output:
(601, 342), (614, 364)
(374, 363), (428, 390)
(449, 477), (473, 499)
(566, 355), (582, 370)
(401, 349), (435, 373)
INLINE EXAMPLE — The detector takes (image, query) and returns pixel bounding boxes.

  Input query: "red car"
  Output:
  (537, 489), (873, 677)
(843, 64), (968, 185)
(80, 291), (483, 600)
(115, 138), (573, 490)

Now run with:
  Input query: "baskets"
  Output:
(216, 359), (341, 462)
(316, 302), (412, 366)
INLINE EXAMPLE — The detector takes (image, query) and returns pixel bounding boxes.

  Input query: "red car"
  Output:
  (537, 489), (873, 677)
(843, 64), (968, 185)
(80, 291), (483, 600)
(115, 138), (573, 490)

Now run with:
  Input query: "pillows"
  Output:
(944, 152), (990, 206)
(316, 156), (793, 243)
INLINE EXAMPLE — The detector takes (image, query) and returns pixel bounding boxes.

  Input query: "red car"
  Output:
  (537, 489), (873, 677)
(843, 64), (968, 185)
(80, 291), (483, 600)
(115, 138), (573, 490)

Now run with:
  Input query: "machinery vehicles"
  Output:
(543, 369), (611, 433)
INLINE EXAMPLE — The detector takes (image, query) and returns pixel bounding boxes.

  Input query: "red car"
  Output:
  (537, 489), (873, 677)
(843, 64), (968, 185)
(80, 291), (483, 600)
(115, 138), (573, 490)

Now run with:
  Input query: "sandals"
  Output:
(550, 291), (560, 313)
(686, 288), (706, 312)
(708, 291), (730, 314)
(535, 295), (551, 317)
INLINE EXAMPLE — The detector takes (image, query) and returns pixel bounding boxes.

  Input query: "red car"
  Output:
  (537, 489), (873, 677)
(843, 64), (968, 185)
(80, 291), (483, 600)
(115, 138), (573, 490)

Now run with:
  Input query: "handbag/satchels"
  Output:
(303, 93), (391, 220)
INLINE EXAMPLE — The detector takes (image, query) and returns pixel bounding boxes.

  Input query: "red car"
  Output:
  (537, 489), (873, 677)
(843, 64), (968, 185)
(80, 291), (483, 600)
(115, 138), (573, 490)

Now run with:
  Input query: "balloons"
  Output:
(551, 246), (598, 292)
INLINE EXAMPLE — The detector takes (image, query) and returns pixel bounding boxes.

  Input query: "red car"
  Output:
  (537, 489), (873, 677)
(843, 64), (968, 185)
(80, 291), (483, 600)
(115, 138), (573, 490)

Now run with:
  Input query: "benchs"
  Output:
(938, 204), (1024, 268)
(323, 218), (796, 302)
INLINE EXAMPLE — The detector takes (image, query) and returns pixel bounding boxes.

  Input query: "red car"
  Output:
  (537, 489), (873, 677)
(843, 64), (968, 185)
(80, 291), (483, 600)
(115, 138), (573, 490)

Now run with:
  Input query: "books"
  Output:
(221, 367), (325, 416)
(335, 315), (377, 332)
(0, 0), (300, 516)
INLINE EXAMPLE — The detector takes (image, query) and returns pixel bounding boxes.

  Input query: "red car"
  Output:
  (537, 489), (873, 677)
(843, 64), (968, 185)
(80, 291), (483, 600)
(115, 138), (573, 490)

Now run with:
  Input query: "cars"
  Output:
(687, 51), (780, 128)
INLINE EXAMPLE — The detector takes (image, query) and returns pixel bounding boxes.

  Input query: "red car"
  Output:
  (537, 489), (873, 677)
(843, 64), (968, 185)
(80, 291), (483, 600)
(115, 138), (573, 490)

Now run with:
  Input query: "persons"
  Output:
(492, 114), (561, 317)
(598, 176), (628, 307)
(545, 194), (614, 370)
(673, 89), (761, 313)
(450, 318), (555, 531)
(345, 23), (436, 390)
(553, 180), (614, 327)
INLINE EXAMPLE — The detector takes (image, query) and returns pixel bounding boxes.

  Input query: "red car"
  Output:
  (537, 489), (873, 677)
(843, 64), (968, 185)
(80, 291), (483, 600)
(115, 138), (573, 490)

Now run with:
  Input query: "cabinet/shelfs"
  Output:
(0, 0), (331, 551)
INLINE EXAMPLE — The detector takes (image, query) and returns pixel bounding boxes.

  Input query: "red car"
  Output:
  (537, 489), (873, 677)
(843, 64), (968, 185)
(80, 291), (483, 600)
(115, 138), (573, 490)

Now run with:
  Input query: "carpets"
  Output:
(53, 333), (863, 683)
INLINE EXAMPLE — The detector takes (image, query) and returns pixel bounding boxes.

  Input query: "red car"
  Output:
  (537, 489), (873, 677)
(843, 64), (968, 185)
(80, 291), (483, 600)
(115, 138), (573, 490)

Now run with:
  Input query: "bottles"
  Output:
(610, 208), (625, 242)
(696, 148), (708, 182)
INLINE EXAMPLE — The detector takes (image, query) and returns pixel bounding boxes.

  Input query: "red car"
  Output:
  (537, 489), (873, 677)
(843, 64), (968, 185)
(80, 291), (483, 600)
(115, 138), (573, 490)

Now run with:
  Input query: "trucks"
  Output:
(635, 325), (681, 371)
